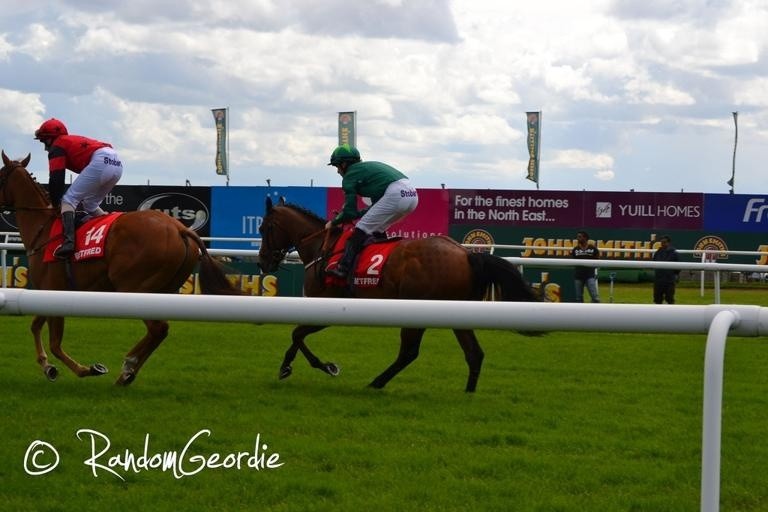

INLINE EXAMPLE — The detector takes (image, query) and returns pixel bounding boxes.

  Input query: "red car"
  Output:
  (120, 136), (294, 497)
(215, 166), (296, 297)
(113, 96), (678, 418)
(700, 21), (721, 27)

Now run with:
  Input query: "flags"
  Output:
(526, 111), (539, 183)
(338, 112), (355, 146)
(211, 108), (226, 175)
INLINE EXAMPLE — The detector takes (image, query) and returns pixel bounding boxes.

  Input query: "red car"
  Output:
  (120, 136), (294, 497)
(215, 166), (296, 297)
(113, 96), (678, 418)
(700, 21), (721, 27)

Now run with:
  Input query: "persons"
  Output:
(34, 120), (123, 259)
(571, 231), (600, 302)
(324, 145), (419, 276)
(653, 234), (678, 304)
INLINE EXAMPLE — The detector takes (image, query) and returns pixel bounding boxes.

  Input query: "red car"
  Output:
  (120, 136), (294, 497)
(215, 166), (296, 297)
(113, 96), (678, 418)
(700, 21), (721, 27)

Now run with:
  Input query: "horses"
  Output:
(256, 197), (546, 392)
(0, 150), (248, 387)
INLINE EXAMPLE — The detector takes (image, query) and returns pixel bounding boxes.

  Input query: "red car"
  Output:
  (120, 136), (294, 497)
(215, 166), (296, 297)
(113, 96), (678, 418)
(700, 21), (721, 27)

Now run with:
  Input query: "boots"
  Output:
(55, 211), (75, 256)
(327, 240), (363, 278)
(373, 231), (386, 239)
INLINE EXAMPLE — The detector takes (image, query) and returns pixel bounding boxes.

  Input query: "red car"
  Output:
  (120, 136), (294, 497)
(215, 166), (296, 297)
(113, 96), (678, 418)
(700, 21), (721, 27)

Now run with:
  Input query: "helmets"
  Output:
(34, 118), (68, 140)
(327, 144), (360, 165)
